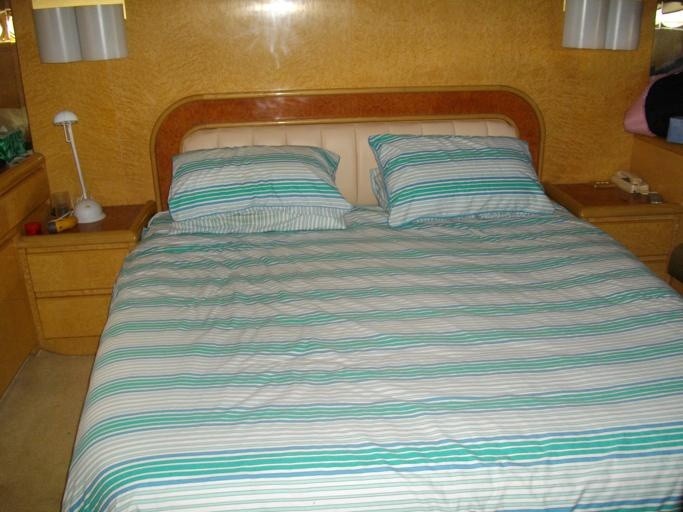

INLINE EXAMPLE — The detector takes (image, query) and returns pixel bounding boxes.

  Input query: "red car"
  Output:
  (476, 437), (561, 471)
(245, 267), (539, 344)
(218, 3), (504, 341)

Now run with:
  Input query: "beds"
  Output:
(62, 87), (682, 512)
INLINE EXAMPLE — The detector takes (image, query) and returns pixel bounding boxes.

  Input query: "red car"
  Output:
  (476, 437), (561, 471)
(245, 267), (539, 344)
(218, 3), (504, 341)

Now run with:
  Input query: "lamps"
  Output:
(54, 110), (106, 225)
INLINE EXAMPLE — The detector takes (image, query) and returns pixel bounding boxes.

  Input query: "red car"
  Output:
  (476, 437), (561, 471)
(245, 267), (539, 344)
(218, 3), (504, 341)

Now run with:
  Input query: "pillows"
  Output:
(364, 134), (560, 238)
(166, 139), (355, 240)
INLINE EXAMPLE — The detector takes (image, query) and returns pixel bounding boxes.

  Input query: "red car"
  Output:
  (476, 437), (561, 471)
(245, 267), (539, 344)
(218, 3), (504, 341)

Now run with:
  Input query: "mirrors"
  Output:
(649, 0), (683, 75)
(0, 0), (34, 174)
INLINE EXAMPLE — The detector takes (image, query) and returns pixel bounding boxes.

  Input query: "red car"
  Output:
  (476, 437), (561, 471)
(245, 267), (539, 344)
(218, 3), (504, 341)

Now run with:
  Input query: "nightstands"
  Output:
(11, 199), (157, 356)
(541, 179), (681, 283)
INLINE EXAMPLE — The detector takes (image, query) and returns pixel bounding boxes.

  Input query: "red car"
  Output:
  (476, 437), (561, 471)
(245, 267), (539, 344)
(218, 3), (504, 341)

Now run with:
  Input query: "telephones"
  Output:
(610, 170), (650, 195)
